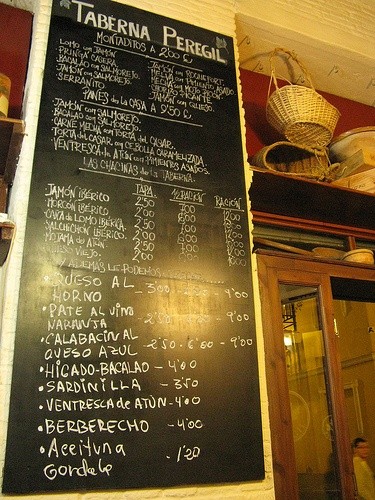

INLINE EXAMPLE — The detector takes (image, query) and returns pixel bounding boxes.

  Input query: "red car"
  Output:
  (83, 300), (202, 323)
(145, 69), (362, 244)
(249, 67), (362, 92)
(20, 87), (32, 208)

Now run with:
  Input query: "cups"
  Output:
(0, 74), (10, 117)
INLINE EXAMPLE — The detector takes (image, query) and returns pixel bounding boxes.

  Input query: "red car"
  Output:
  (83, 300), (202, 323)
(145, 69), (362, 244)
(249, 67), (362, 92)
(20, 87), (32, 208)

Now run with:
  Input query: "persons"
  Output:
(352, 437), (375, 500)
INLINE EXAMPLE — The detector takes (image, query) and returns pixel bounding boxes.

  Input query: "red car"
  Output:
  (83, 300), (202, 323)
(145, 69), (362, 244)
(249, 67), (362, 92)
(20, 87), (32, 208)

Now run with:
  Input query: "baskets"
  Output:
(251, 141), (332, 180)
(265, 48), (340, 152)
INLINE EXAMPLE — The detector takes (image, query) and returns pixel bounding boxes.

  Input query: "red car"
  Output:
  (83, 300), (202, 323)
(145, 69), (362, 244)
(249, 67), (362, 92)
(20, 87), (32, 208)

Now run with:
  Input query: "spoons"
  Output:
(253, 233), (344, 259)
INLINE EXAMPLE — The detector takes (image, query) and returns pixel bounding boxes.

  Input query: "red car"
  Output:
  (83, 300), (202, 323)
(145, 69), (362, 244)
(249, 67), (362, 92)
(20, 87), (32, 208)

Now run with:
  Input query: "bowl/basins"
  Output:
(342, 248), (374, 265)
(328, 126), (375, 174)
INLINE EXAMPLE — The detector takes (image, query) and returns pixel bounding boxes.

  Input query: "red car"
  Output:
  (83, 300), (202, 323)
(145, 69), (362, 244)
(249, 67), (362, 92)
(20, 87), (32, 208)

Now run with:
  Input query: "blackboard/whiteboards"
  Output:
(0, 0), (268, 496)
